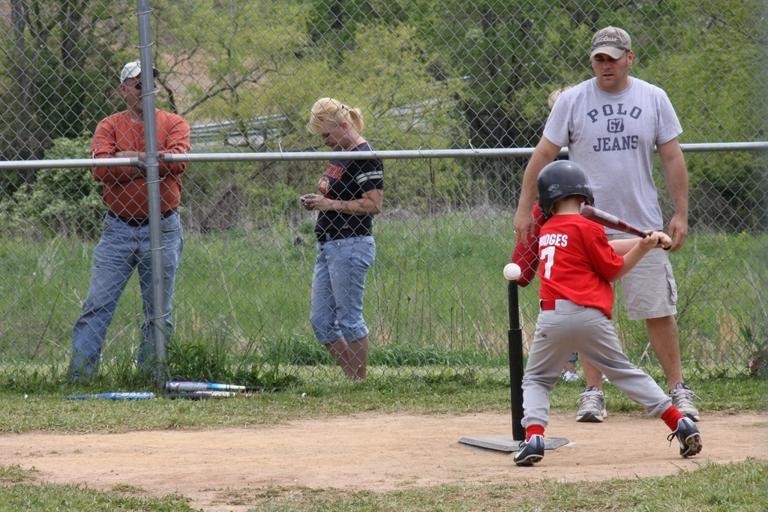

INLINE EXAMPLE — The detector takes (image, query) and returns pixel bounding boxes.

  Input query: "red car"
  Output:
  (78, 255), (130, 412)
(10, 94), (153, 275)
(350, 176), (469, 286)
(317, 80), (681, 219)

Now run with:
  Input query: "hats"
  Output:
(589, 24), (632, 62)
(119, 61), (160, 84)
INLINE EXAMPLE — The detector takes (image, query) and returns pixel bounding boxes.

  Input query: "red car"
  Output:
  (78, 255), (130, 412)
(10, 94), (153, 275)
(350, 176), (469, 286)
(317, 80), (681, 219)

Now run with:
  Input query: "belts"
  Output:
(107, 206), (177, 228)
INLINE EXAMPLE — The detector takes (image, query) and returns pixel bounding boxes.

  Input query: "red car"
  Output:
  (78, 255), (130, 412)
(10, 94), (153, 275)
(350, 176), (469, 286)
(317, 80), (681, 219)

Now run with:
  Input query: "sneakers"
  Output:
(575, 384), (607, 422)
(513, 434), (546, 465)
(673, 417), (703, 456)
(668, 382), (700, 421)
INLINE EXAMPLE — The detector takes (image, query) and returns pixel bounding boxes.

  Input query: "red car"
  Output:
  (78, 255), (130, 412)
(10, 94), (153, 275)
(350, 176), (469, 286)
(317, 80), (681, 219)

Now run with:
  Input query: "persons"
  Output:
(67, 63), (190, 384)
(514, 161), (703, 466)
(511, 27), (699, 421)
(299, 99), (385, 380)
(547, 87), (607, 383)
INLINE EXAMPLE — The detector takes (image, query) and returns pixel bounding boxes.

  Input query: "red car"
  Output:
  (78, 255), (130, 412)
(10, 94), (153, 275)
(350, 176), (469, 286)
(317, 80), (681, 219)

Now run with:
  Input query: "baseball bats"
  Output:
(165, 381), (306, 400)
(582, 206), (672, 250)
(23, 392), (155, 402)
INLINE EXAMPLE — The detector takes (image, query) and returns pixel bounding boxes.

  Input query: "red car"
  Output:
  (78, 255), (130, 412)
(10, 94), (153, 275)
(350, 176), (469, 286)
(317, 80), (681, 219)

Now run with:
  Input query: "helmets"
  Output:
(536, 159), (596, 215)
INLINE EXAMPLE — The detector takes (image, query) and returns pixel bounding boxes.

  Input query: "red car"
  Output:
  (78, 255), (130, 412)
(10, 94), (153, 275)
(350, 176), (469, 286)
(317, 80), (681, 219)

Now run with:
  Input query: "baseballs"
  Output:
(502, 264), (521, 280)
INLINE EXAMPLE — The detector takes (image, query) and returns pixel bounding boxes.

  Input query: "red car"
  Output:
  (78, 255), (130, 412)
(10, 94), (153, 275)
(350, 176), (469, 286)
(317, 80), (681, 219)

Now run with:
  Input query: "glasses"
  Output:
(134, 81), (156, 90)
